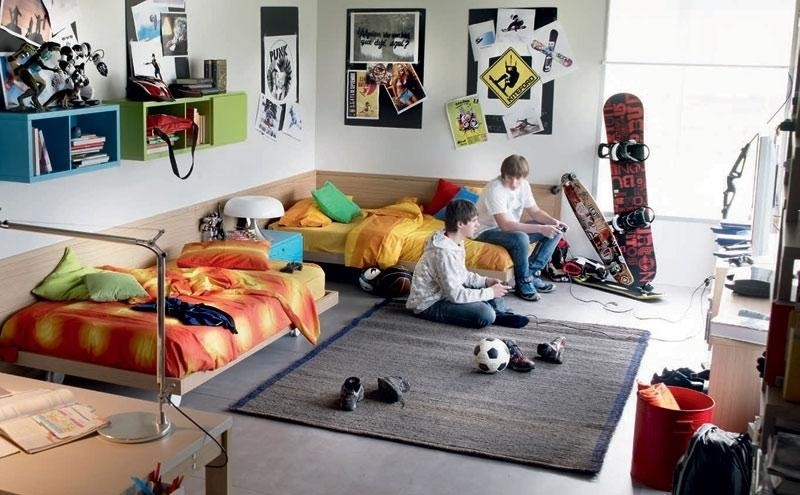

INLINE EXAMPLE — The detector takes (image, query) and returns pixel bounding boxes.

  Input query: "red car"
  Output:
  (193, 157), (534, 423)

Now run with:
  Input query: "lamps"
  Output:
(222, 194), (287, 243)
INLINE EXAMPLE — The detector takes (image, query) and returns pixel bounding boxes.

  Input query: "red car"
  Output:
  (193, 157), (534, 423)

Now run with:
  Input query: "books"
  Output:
(186, 107), (206, 147)
(38, 130), (53, 173)
(32, 127), (41, 176)
(174, 76), (220, 97)
(0, 381), (114, 457)
(146, 133), (180, 155)
(70, 134), (110, 170)
(203, 58), (227, 94)
(0, 437), (20, 461)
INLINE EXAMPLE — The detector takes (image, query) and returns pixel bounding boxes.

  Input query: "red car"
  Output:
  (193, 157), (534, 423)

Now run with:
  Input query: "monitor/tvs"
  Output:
(750, 123), (775, 259)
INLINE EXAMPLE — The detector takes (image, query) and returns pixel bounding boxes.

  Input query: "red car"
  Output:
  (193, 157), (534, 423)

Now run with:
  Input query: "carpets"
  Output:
(228, 295), (651, 475)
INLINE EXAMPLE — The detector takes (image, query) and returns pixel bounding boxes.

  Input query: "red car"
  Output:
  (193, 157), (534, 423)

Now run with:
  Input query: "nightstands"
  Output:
(226, 230), (308, 267)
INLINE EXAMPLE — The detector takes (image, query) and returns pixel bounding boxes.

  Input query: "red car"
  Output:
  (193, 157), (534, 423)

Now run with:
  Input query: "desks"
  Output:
(699, 223), (767, 433)
(1, 373), (235, 494)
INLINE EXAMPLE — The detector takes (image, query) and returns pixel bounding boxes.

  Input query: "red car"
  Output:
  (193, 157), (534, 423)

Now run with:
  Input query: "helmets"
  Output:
(128, 75), (175, 102)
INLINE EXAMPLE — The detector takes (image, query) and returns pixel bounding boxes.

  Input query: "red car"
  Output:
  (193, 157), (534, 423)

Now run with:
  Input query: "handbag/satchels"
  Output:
(147, 114), (193, 136)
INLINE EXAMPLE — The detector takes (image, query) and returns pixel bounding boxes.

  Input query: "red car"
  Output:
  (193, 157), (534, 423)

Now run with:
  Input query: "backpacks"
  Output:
(673, 423), (752, 495)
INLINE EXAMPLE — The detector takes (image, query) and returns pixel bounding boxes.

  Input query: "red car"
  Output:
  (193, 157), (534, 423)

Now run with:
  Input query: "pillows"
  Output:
(278, 192), (356, 228)
(435, 186), (482, 221)
(180, 239), (271, 271)
(32, 248), (112, 303)
(425, 179), (456, 214)
(314, 181), (361, 226)
(80, 270), (146, 305)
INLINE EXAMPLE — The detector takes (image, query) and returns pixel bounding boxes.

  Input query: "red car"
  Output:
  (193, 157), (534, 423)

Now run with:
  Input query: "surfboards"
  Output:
(510, 127), (529, 135)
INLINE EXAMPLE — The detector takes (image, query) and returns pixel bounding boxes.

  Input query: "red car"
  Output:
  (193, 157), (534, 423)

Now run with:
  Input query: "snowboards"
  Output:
(501, 25), (528, 33)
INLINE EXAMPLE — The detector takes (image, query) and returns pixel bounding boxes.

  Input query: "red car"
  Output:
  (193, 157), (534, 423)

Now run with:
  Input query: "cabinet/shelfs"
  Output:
(756, 96), (800, 495)
(120, 91), (249, 161)
(2, 102), (122, 185)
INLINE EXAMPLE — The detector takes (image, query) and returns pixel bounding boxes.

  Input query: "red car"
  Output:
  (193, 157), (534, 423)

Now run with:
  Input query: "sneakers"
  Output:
(378, 375), (411, 405)
(537, 336), (566, 364)
(532, 271), (555, 293)
(340, 377), (364, 411)
(651, 368), (710, 391)
(516, 278), (540, 300)
(503, 340), (535, 372)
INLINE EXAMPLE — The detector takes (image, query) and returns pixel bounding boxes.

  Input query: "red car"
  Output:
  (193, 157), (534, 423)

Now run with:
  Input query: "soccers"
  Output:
(359, 267), (381, 293)
(474, 337), (510, 374)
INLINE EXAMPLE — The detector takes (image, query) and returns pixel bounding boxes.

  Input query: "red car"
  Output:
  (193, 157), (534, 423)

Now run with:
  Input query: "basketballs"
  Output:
(380, 265), (412, 297)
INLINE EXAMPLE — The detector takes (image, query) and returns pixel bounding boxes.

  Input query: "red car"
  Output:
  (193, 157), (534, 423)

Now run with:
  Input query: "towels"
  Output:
(135, 294), (239, 335)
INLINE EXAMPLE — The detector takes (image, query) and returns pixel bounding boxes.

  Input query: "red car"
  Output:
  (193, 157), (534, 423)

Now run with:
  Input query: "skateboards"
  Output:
(552, 171), (635, 286)
(570, 274), (665, 301)
(603, 93), (656, 284)
(530, 28), (571, 73)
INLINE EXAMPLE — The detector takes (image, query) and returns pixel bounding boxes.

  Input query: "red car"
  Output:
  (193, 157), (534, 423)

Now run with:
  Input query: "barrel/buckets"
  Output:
(629, 382), (718, 494)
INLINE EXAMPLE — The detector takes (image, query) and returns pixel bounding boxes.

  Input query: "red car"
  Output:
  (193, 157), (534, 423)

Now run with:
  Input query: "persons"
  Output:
(403, 200), (532, 329)
(358, 101), (375, 113)
(512, 117), (537, 130)
(388, 63), (417, 106)
(507, 14), (524, 32)
(495, 66), (520, 92)
(469, 154), (572, 303)
(143, 52), (163, 79)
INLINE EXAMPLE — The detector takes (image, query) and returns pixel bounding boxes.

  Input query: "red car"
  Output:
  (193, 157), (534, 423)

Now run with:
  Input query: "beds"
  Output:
(1, 247), (340, 402)
(273, 189), (557, 291)
